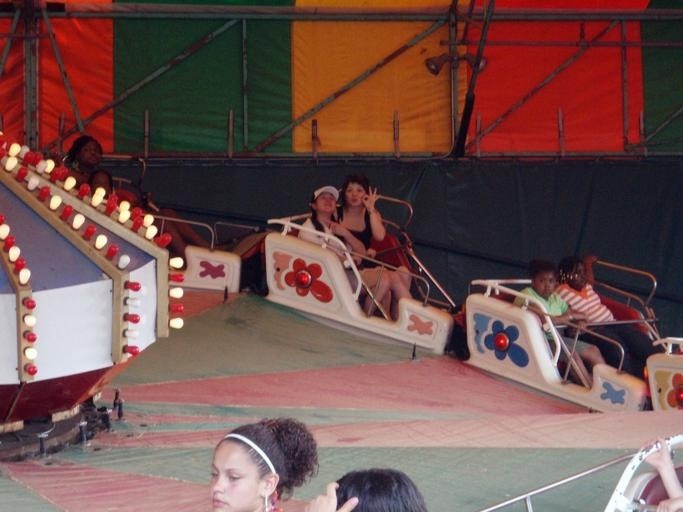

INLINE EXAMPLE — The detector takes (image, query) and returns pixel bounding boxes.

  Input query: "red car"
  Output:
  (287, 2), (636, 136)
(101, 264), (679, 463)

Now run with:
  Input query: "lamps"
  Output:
(423, 52), (492, 75)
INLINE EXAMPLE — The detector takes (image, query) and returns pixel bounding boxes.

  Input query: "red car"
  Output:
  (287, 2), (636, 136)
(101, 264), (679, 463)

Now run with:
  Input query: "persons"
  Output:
(210, 417), (319, 512)
(61, 134), (235, 271)
(330, 175), (416, 321)
(639, 434), (682, 512)
(297, 184), (393, 324)
(304, 466), (429, 512)
(552, 253), (667, 377)
(513, 258), (607, 390)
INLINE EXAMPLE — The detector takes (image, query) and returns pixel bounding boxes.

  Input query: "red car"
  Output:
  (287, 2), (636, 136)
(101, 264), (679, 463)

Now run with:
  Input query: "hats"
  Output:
(311, 185), (339, 203)
